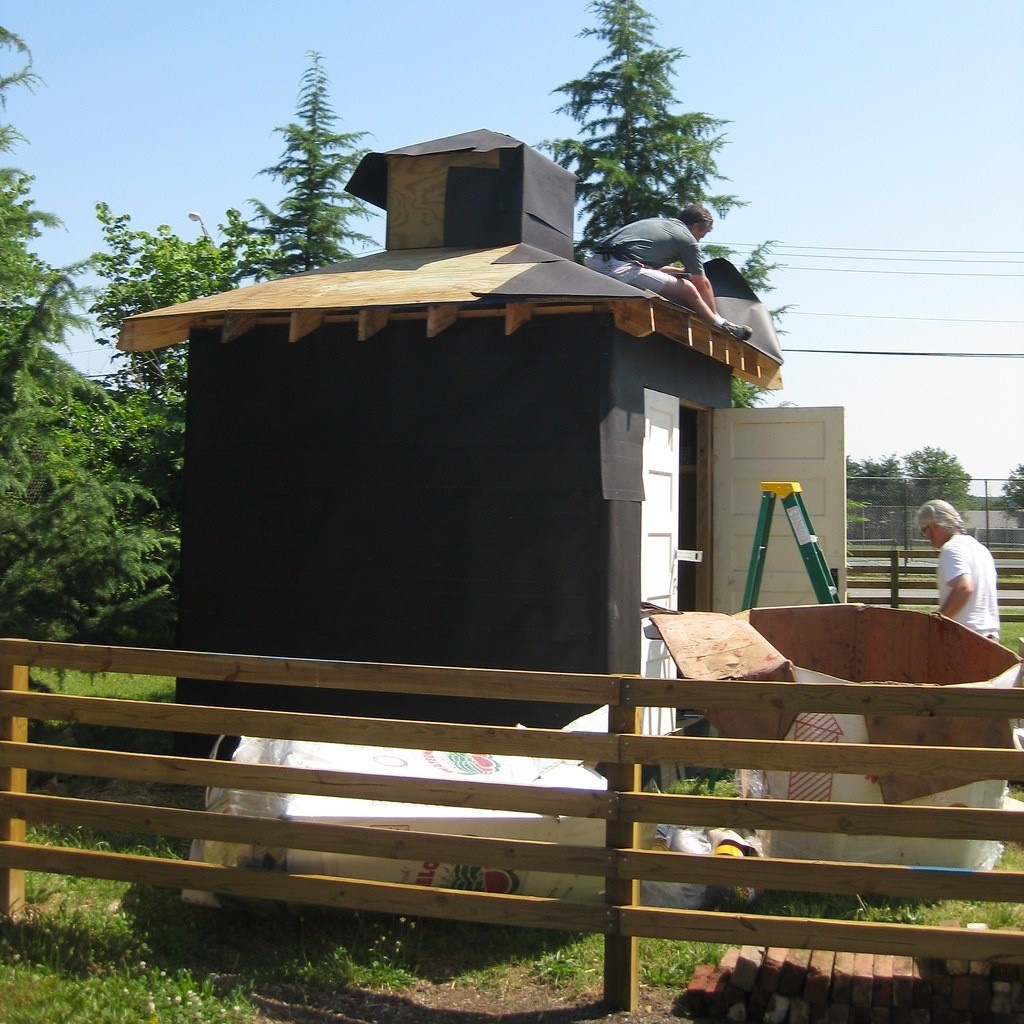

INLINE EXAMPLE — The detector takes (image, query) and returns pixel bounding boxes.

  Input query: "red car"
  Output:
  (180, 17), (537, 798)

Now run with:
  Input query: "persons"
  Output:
(919, 500), (1000, 643)
(590, 203), (753, 340)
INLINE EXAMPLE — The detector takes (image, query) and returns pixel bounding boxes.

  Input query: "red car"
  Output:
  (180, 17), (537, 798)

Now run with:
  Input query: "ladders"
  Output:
(741, 481), (841, 612)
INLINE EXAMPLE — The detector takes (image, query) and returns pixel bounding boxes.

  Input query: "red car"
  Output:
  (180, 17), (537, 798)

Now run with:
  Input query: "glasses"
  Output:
(918, 522), (932, 538)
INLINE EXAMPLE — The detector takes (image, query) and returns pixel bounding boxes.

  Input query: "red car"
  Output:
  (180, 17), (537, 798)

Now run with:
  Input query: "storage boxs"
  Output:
(645, 602), (1024, 870)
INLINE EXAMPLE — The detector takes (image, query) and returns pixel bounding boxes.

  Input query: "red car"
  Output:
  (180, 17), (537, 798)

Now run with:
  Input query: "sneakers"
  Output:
(722, 322), (753, 341)
